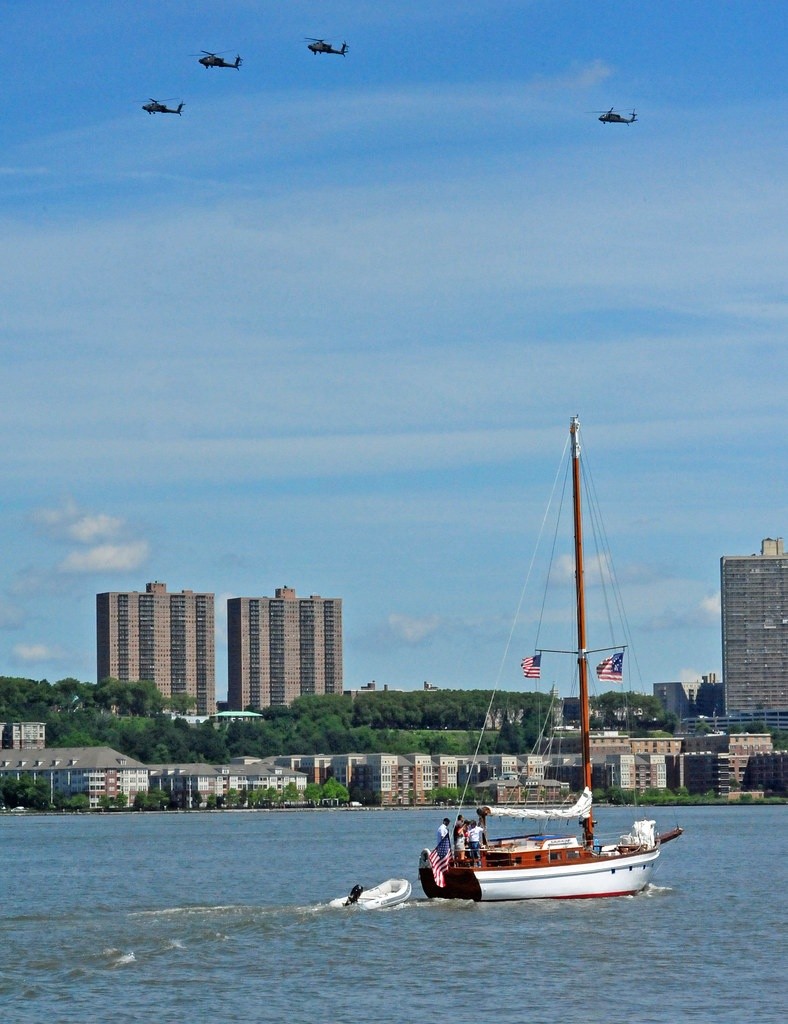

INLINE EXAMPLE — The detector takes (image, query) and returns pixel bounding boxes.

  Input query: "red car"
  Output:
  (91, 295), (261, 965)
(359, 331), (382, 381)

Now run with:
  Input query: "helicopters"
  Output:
(189, 50), (244, 70)
(303, 37), (349, 58)
(585, 108), (638, 126)
(136, 98), (186, 116)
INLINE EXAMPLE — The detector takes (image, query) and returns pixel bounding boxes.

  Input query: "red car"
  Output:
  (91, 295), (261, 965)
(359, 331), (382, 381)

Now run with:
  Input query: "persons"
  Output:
(435, 817), (450, 845)
(453, 815), (485, 868)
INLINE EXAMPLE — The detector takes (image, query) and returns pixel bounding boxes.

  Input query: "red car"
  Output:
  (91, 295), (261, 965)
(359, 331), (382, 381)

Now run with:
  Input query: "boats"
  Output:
(329, 879), (412, 912)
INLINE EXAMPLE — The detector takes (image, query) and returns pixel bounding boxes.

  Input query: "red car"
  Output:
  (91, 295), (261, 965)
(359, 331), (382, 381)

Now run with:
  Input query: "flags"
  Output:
(429, 832), (452, 888)
(520, 654), (542, 679)
(594, 652), (624, 682)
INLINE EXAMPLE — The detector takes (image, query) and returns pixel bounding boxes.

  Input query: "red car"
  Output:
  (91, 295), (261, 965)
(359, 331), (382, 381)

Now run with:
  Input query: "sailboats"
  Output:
(419, 414), (683, 904)
